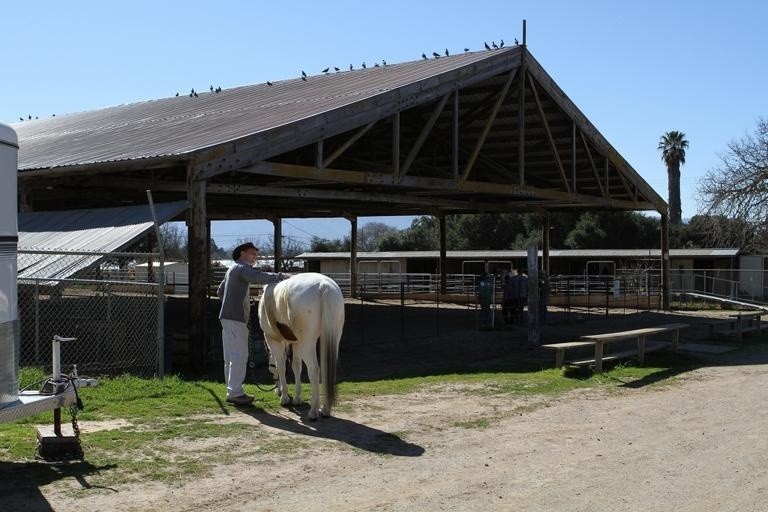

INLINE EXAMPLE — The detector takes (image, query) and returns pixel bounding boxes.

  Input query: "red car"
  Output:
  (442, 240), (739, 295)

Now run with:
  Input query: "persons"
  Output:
(478, 266), (550, 331)
(217, 242), (293, 405)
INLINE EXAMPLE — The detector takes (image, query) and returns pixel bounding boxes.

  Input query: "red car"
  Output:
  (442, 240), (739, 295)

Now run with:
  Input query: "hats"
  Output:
(233, 242), (258, 262)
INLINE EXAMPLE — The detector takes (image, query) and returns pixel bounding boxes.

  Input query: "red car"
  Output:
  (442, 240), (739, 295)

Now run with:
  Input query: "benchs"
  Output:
(542, 322), (690, 373)
(702, 311), (768, 345)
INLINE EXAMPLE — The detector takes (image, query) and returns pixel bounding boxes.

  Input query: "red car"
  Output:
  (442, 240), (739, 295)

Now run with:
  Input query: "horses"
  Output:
(256, 270), (348, 421)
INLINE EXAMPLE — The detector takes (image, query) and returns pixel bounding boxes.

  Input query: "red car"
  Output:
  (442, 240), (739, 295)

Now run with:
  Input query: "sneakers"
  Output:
(226, 394), (255, 405)
